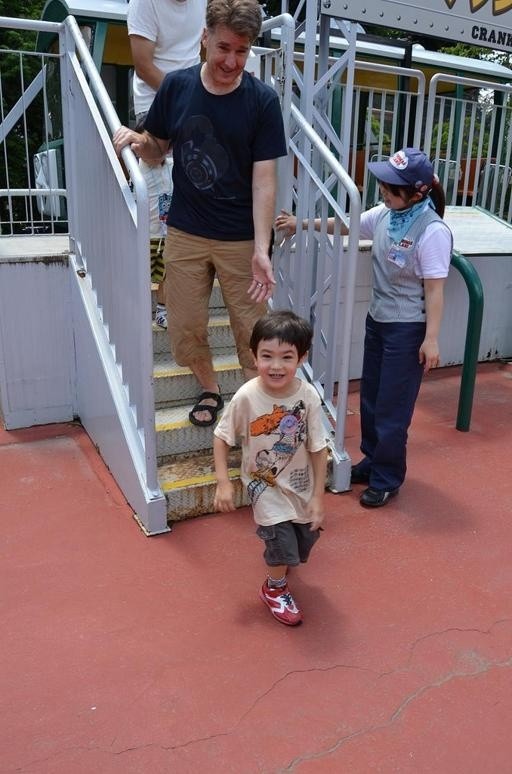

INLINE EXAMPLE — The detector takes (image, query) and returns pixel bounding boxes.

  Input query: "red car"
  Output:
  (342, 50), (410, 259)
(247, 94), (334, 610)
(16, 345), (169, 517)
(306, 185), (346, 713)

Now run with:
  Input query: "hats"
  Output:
(367, 149), (434, 196)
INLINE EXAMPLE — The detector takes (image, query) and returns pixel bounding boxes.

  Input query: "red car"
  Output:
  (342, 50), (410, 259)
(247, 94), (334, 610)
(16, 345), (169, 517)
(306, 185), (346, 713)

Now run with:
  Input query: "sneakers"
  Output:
(259, 577), (304, 627)
(359, 485), (399, 507)
(352, 458), (369, 481)
(155, 301), (172, 328)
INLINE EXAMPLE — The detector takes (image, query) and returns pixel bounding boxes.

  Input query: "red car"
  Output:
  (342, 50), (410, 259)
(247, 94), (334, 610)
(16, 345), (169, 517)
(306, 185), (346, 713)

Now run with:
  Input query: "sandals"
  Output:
(188, 389), (224, 426)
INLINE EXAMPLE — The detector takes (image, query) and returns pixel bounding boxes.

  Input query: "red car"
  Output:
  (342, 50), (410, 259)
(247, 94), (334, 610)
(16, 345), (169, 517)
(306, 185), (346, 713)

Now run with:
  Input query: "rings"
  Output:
(257, 283), (263, 286)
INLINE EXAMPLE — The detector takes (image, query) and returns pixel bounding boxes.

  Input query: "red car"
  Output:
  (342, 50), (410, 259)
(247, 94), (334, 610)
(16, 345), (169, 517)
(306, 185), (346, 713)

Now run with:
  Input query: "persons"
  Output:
(126, 0), (209, 125)
(127, 116), (175, 329)
(214, 311), (328, 625)
(274, 148), (453, 507)
(112, 1), (288, 427)
